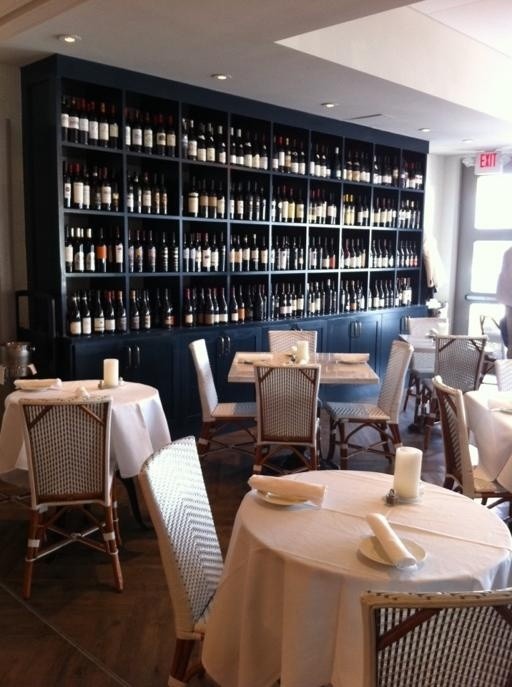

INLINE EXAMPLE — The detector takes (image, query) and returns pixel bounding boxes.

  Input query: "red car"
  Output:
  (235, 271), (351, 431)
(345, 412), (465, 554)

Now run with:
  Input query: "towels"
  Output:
(12, 378), (63, 391)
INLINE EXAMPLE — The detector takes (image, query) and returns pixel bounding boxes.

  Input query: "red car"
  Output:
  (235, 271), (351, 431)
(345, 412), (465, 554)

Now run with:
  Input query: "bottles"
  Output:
(271, 281), (305, 320)
(62, 160), (121, 211)
(403, 155), (425, 190)
(128, 228), (179, 272)
(370, 278), (394, 309)
(231, 178), (268, 220)
(68, 285), (127, 336)
(184, 173), (226, 219)
(231, 233), (268, 272)
(371, 237), (396, 268)
(127, 166), (168, 214)
(130, 287), (177, 332)
(398, 238), (420, 268)
(306, 280), (339, 316)
(342, 147), (371, 184)
(309, 235), (337, 269)
(183, 228), (227, 271)
(273, 233), (305, 271)
(126, 106), (178, 158)
(342, 192), (370, 226)
(274, 133), (307, 175)
(64, 222), (124, 272)
(184, 286), (229, 327)
(341, 276), (367, 312)
(310, 185), (340, 224)
(342, 236), (367, 268)
(399, 197), (421, 229)
(229, 123), (269, 170)
(62, 93), (121, 148)
(373, 193), (398, 227)
(397, 276), (416, 306)
(374, 152), (399, 187)
(273, 181), (305, 222)
(183, 116), (227, 163)
(229, 282), (267, 323)
(309, 141), (342, 179)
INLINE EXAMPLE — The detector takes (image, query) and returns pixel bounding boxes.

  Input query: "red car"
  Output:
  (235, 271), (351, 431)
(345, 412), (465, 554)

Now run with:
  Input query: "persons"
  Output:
(496, 245), (512, 359)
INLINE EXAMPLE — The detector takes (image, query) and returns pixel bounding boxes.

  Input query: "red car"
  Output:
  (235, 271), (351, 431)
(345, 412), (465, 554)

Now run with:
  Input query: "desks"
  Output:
(5, 382), (173, 538)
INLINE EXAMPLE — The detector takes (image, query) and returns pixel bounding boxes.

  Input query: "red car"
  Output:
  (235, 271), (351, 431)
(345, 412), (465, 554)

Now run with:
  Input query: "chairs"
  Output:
(20, 398), (126, 598)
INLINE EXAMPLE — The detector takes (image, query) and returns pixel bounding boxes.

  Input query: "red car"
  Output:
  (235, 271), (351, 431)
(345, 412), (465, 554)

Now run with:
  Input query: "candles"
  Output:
(103, 358), (120, 387)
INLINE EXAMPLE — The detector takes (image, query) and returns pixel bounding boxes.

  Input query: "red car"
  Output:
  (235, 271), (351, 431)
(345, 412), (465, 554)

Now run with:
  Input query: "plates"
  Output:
(250, 486), (307, 506)
(14, 379), (58, 390)
(333, 353), (369, 363)
(358, 534), (427, 567)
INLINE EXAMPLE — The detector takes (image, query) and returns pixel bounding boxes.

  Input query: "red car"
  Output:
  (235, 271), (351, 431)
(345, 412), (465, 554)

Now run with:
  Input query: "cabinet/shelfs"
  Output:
(20, 54), (430, 417)
